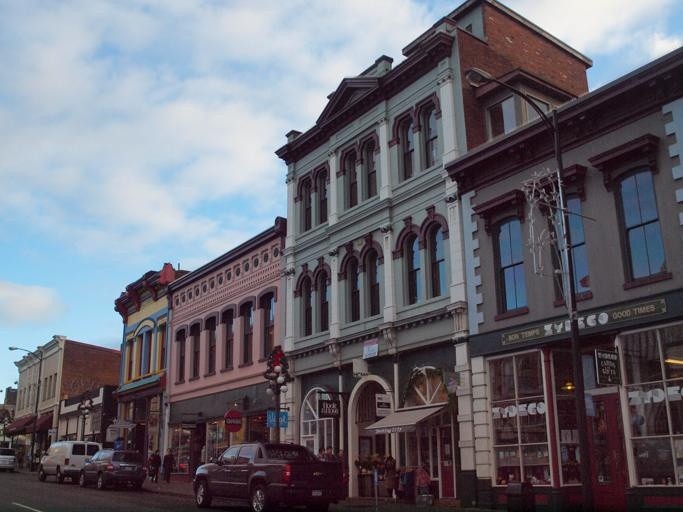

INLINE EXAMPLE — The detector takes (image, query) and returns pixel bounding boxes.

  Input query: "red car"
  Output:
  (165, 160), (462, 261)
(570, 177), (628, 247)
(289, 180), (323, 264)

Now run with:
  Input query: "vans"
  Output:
(37, 441), (103, 484)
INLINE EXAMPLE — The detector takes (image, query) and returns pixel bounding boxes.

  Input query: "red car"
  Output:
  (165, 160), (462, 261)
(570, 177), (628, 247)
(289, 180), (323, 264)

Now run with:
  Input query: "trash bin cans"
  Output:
(360, 473), (375, 497)
(507, 480), (535, 512)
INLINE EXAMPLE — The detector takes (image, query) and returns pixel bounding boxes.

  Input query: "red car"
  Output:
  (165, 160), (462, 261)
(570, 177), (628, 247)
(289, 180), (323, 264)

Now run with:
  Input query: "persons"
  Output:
(317, 446), (350, 505)
(16, 447), (24, 469)
(148, 448), (161, 483)
(163, 447), (176, 483)
(382, 453), (396, 498)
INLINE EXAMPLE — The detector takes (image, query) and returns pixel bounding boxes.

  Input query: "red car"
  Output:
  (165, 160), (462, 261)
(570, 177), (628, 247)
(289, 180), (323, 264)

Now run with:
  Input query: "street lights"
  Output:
(464, 66), (597, 512)
(263, 344), (295, 444)
(9, 347), (43, 472)
(77, 391), (94, 440)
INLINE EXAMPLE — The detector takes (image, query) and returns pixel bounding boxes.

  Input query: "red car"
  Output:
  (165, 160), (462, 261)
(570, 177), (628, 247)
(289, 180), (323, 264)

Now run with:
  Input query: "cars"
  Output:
(0, 448), (17, 471)
(78, 448), (147, 490)
(193, 442), (349, 512)
